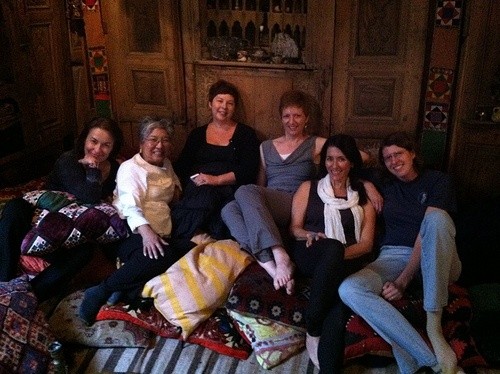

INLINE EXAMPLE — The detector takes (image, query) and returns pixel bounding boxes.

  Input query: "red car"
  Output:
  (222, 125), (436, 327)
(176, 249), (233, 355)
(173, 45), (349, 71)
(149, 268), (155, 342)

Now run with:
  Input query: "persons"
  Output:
(80, 116), (182, 326)
(221, 91), (382, 295)
(0, 116), (123, 300)
(173, 80), (257, 257)
(338, 131), (462, 374)
(291, 135), (375, 374)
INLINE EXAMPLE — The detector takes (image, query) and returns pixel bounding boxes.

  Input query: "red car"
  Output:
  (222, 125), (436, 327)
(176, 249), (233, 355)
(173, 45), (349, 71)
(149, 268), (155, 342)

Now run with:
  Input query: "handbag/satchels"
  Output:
(271, 23), (298, 58)
(202, 31), (251, 60)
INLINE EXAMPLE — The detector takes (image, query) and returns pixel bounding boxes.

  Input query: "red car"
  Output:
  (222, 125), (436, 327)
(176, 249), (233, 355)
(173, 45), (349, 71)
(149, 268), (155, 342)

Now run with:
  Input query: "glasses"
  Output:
(381, 149), (406, 163)
(144, 136), (171, 146)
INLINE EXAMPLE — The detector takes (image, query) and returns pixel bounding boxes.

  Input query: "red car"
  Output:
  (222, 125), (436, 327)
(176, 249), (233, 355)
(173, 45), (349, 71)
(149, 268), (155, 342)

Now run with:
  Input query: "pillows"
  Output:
(48, 261), (488, 365)
